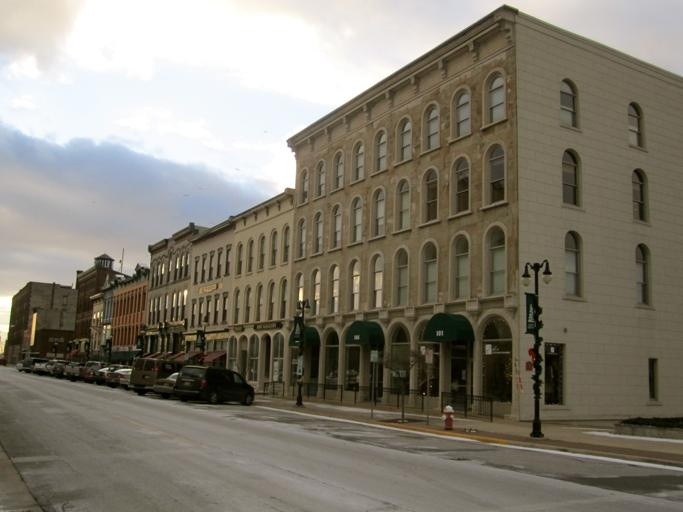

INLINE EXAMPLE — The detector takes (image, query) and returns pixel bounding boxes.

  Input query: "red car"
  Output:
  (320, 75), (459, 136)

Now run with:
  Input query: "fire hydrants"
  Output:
(442, 405), (454, 430)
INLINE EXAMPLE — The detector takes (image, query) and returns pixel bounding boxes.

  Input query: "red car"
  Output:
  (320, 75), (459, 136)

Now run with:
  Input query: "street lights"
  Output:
(194, 316), (209, 366)
(296, 299), (311, 406)
(521, 259), (553, 438)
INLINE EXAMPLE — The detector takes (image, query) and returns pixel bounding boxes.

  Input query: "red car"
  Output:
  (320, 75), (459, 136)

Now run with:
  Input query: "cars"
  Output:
(17, 357), (254, 406)
